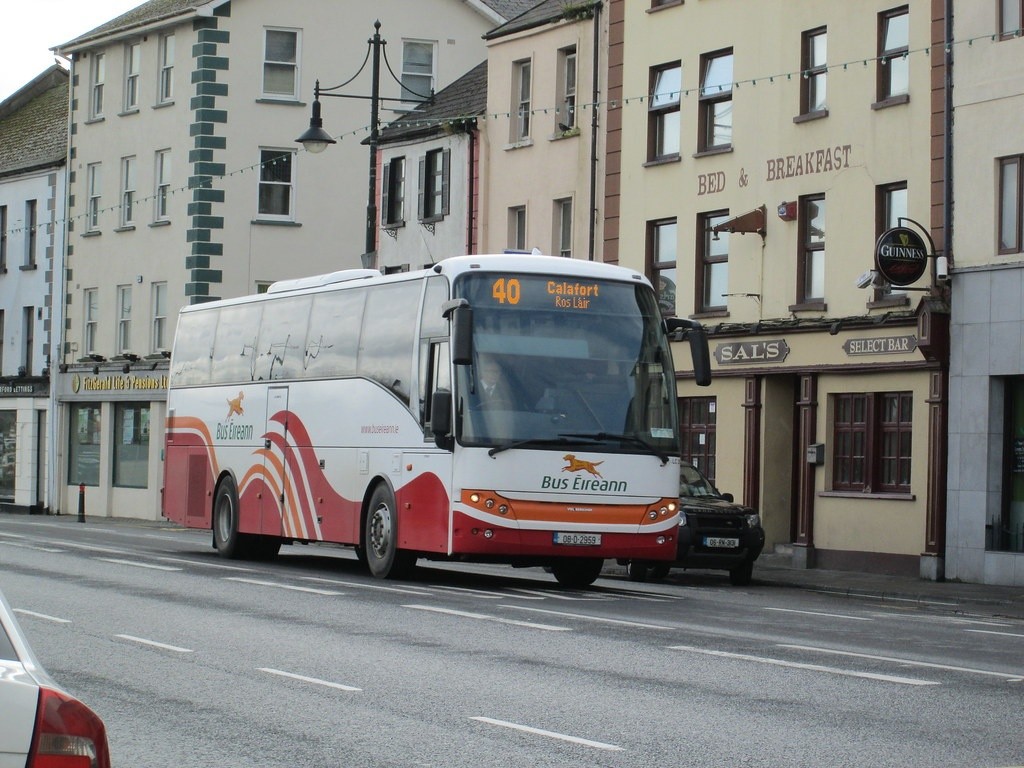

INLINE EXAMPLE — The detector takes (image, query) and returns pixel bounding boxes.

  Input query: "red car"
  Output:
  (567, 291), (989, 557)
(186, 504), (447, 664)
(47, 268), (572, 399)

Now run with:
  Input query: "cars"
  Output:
(1, 593), (114, 768)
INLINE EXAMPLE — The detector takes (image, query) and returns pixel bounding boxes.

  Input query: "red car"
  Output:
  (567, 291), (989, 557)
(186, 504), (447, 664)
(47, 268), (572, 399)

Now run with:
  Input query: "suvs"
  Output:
(624, 459), (767, 586)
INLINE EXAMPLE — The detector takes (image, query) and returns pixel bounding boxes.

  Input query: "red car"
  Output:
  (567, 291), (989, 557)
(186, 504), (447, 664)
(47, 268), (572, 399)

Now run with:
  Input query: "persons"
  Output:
(472, 360), (514, 411)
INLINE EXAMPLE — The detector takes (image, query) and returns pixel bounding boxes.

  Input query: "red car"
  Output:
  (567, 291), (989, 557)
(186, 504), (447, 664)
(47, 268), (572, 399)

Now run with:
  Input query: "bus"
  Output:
(160, 248), (713, 589)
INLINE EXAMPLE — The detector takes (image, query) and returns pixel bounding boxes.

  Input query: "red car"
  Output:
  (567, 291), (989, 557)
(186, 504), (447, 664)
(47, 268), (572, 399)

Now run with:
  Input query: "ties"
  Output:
(486, 387), (493, 397)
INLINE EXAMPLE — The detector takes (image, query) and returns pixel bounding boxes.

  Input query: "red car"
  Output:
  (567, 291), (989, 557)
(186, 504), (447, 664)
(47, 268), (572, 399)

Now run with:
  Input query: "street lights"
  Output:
(294, 17), (437, 268)
